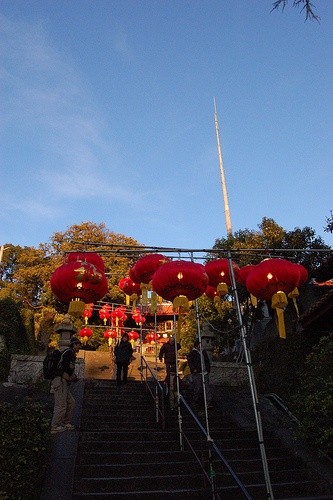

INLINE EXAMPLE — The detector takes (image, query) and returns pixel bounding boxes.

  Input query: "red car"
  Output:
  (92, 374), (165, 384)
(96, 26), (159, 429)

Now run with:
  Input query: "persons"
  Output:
(163, 369), (186, 411)
(159, 337), (181, 382)
(50, 337), (82, 433)
(187, 341), (214, 410)
(114, 333), (133, 387)
(136, 337), (140, 353)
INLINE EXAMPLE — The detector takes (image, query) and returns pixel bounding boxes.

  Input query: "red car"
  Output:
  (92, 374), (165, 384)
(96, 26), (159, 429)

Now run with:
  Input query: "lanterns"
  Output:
(50, 252), (307, 351)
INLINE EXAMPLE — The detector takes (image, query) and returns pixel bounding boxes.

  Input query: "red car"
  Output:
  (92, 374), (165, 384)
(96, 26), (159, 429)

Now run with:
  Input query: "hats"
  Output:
(69, 337), (80, 346)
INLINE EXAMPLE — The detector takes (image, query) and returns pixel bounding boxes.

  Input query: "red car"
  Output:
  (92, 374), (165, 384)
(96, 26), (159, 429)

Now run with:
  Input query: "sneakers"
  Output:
(51, 426), (67, 434)
(64, 424), (78, 430)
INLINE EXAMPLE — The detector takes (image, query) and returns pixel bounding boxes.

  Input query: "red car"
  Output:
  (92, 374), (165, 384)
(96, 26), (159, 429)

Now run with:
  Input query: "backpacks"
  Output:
(43, 348), (71, 380)
